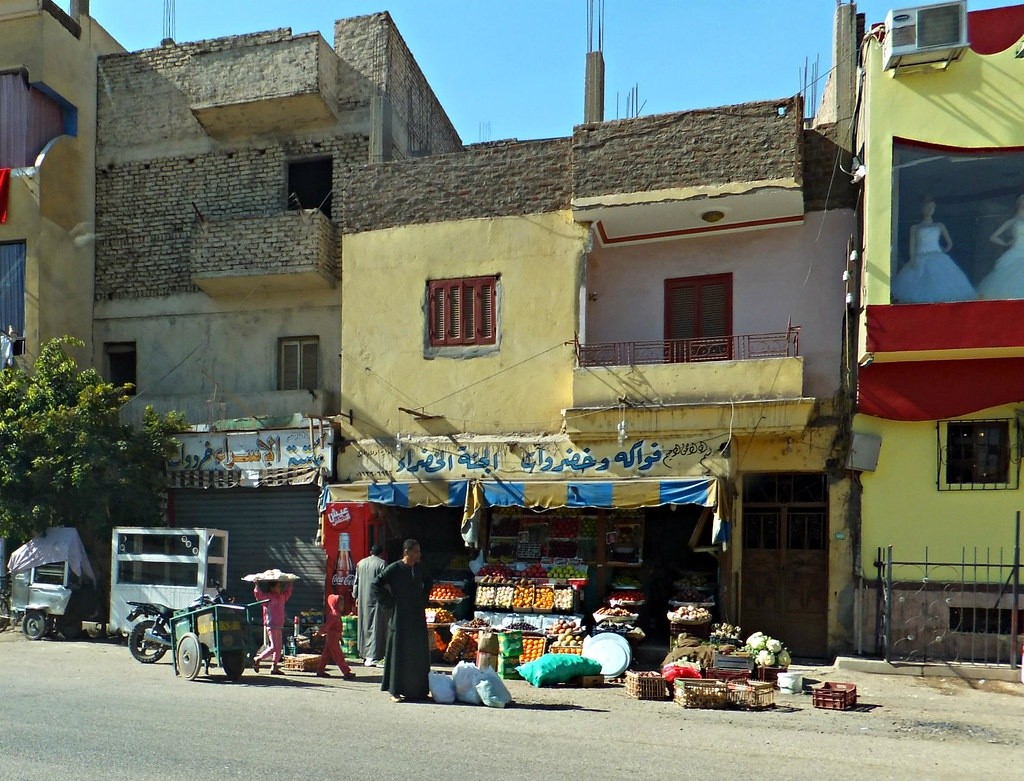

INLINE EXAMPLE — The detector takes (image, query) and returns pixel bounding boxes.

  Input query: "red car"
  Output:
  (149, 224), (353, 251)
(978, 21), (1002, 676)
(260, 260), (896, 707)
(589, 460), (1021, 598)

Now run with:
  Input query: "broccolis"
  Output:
(745, 631), (791, 667)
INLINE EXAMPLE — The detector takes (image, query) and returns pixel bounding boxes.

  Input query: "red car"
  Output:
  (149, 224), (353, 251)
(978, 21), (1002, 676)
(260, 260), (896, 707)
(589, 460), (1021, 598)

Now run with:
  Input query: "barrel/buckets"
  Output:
(777, 672), (803, 694)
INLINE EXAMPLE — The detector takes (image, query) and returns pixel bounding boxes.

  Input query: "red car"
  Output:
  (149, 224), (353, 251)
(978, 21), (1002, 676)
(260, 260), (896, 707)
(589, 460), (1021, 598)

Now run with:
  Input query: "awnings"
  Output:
(316, 471), (731, 553)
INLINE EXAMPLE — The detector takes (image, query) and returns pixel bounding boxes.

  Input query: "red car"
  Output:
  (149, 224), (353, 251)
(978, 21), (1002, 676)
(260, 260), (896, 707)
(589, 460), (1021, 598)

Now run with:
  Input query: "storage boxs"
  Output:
(710, 637), (738, 647)
(475, 651), (496, 672)
(576, 675), (604, 687)
(523, 636), (549, 656)
(497, 654), (522, 680)
(755, 666), (787, 689)
(341, 616), (357, 639)
(670, 624), (706, 636)
(340, 638), (358, 659)
(812, 682), (857, 710)
(705, 668), (752, 680)
(497, 631), (524, 657)
(474, 507), (646, 637)
(674, 678), (726, 709)
(727, 677), (776, 711)
(625, 670), (667, 700)
(478, 630), (498, 655)
(301, 611), (322, 624)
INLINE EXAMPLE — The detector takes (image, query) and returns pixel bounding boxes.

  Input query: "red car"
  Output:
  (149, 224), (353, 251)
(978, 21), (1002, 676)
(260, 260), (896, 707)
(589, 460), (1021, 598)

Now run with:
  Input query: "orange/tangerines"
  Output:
(551, 647), (582, 655)
(428, 582), (465, 600)
(519, 639), (544, 663)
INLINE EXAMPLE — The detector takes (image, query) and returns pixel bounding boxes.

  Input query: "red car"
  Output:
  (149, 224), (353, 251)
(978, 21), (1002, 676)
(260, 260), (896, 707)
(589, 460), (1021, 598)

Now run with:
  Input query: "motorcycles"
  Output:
(126, 579), (238, 663)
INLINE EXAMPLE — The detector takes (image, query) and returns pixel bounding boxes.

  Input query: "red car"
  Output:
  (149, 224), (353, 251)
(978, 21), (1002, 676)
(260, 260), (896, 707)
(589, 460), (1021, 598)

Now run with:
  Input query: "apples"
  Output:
(607, 591), (646, 601)
(462, 633), (479, 659)
(549, 517), (579, 539)
(476, 561), (586, 647)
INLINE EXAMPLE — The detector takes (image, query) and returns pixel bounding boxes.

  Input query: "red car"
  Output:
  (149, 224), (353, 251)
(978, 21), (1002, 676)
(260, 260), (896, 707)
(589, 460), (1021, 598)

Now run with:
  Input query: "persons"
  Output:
(976, 193), (1024, 299)
(352, 544), (393, 667)
(893, 194), (979, 303)
(370, 538), (430, 703)
(317, 594), (356, 679)
(253, 578), (293, 674)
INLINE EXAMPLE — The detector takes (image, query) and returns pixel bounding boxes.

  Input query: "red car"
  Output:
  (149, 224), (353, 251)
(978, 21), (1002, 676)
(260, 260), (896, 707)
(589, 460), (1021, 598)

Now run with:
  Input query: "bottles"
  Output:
(331, 532), (358, 615)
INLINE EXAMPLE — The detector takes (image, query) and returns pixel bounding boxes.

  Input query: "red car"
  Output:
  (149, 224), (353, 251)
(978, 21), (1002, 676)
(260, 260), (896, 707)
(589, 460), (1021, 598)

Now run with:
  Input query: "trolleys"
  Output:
(170, 599), (272, 682)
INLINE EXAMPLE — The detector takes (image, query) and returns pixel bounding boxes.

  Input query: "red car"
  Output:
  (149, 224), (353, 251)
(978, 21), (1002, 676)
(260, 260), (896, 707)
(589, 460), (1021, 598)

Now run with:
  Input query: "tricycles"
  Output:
(0, 527), (94, 641)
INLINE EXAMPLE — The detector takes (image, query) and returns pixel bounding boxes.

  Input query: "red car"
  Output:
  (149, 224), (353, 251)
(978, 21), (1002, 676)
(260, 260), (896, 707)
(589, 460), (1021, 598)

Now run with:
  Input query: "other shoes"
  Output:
(405, 696), (426, 703)
(317, 672), (330, 677)
(344, 673), (355, 680)
(364, 660), (376, 667)
(253, 657), (259, 673)
(271, 665), (284, 675)
(388, 696), (402, 702)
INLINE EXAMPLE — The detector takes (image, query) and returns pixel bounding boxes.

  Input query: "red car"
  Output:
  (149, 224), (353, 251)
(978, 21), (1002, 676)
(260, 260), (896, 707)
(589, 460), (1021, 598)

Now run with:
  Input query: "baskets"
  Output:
(706, 665), (788, 689)
(812, 682), (857, 710)
(728, 680), (774, 708)
(626, 670), (666, 699)
(673, 678), (730, 708)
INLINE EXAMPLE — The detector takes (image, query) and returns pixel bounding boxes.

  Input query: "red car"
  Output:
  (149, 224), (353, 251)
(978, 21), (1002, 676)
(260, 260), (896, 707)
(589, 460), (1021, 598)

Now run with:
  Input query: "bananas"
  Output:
(486, 555), (514, 564)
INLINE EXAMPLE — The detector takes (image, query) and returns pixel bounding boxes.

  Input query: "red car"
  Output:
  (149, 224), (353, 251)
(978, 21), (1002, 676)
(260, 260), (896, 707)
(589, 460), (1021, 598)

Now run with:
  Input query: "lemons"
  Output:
(582, 518), (610, 537)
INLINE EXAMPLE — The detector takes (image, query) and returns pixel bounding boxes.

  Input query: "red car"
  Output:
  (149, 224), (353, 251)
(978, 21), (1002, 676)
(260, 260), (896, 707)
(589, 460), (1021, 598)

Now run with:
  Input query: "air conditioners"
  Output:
(882, 0), (969, 72)
